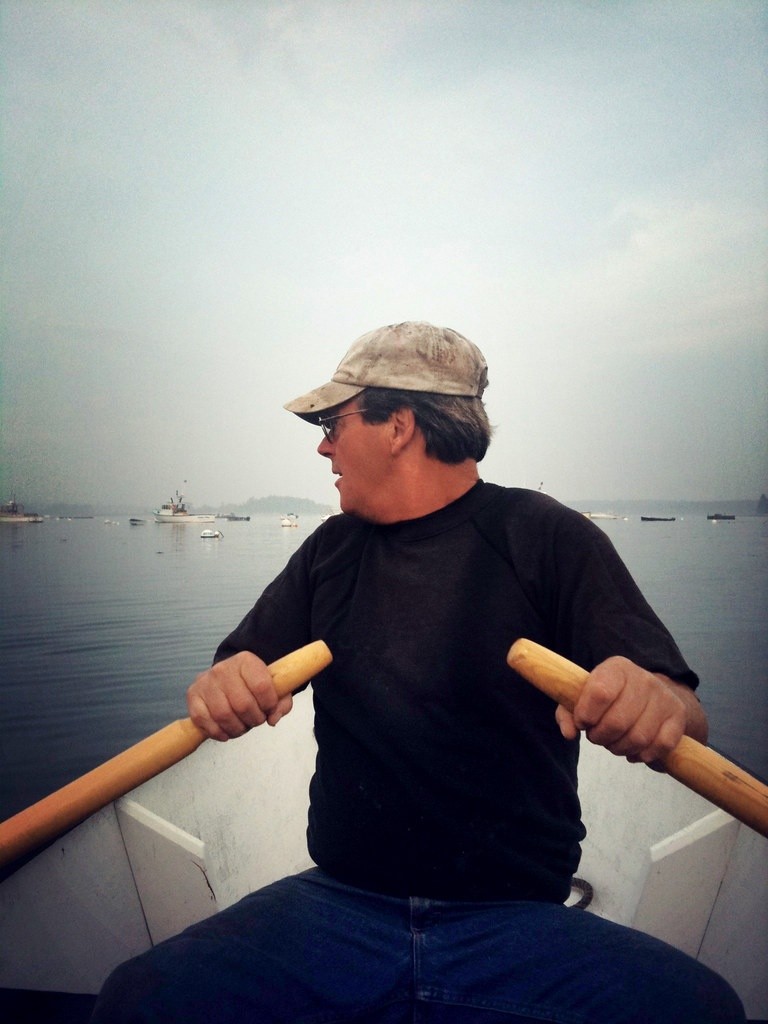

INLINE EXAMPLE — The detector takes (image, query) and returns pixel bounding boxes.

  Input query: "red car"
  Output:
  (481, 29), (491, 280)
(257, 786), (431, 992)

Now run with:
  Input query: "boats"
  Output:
(129, 518), (150, 526)
(282, 515), (298, 528)
(706, 512), (736, 520)
(2, 671), (766, 1021)
(151, 480), (250, 523)
(104, 520), (120, 525)
(640, 515), (676, 522)
(0, 490), (44, 523)
(201, 528), (223, 539)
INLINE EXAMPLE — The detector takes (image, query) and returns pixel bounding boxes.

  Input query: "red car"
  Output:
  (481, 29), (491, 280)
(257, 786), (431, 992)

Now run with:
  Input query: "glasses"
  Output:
(319, 407), (371, 445)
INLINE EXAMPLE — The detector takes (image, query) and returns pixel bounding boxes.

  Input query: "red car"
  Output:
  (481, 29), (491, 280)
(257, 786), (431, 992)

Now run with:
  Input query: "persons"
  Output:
(95, 321), (748, 1024)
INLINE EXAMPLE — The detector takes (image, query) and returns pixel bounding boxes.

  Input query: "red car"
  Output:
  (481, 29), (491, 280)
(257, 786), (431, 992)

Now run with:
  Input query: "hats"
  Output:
(287, 324), (492, 426)
